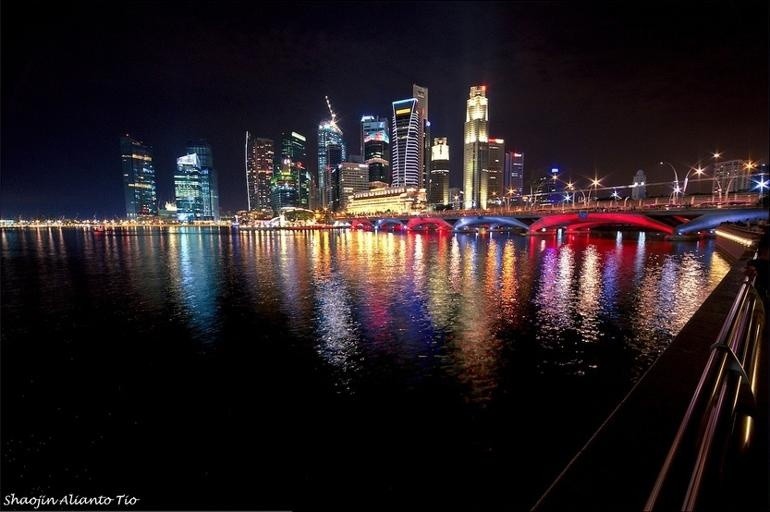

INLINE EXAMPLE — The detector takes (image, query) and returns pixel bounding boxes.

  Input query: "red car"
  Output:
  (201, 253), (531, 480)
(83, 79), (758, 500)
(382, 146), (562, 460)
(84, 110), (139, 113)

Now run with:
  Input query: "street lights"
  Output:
(658, 154), (753, 201)
(547, 174), (598, 208)
(493, 188), (523, 210)
